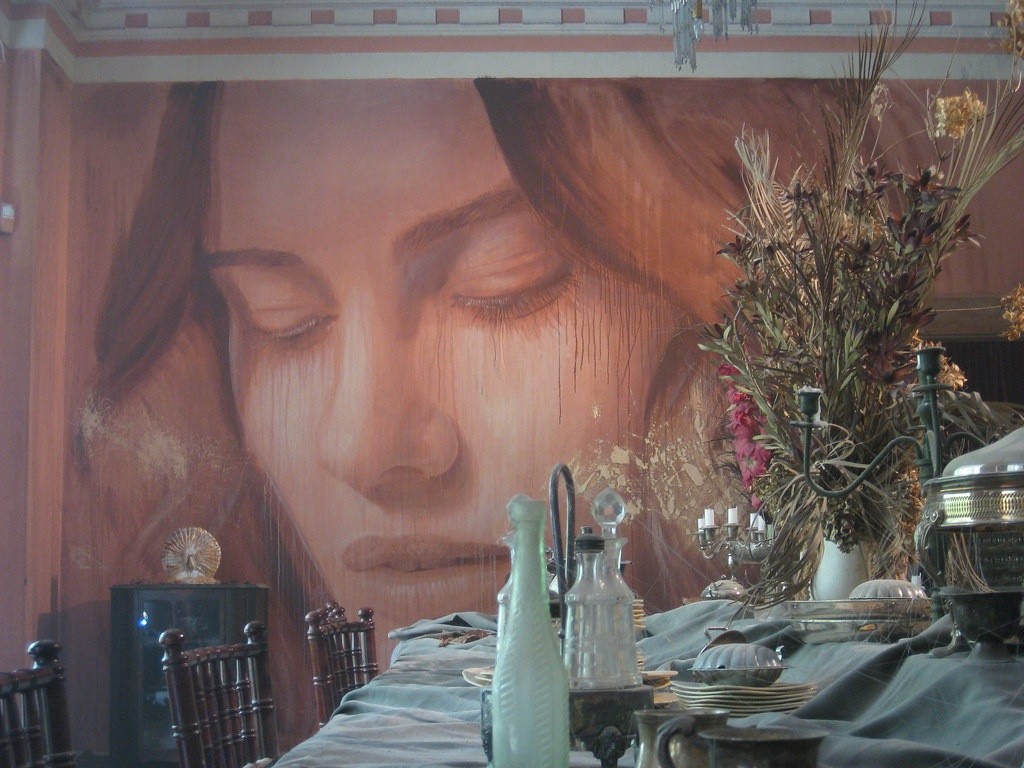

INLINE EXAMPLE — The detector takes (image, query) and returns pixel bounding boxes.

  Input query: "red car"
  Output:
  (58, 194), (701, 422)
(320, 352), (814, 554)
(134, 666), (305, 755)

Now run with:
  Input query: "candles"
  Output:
(727, 506), (738, 523)
(758, 515), (765, 530)
(704, 507), (715, 525)
(750, 512), (758, 526)
(698, 518), (704, 529)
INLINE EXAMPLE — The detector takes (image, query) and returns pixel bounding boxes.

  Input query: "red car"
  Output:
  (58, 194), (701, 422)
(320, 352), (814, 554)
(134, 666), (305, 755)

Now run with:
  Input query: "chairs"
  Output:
(159, 620), (280, 768)
(306, 600), (378, 731)
(0, 638), (75, 768)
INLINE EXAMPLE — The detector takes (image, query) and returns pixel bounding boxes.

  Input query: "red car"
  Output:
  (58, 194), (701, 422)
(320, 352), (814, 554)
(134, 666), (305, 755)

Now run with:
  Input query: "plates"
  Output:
(462, 665), (680, 706)
(633, 598), (680, 671)
(669, 680), (818, 718)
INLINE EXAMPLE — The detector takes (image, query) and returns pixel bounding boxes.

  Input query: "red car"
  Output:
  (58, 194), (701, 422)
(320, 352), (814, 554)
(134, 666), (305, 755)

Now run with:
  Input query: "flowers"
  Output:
(697, 0), (1022, 609)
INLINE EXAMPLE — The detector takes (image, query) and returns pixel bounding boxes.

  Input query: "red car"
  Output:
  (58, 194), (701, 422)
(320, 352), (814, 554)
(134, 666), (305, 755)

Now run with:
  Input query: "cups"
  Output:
(656, 716), (828, 768)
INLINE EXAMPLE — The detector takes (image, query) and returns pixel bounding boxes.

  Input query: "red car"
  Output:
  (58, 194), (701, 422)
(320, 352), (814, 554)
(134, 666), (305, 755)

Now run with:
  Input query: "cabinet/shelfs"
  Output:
(109, 585), (267, 768)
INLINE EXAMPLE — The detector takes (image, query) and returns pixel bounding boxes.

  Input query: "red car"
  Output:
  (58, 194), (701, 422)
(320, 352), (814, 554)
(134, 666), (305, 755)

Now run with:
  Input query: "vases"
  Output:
(811, 538), (867, 599)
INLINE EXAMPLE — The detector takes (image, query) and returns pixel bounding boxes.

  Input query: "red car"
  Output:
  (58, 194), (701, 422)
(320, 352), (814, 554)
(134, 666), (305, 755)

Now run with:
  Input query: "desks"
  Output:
(271, 601), (1023, 768)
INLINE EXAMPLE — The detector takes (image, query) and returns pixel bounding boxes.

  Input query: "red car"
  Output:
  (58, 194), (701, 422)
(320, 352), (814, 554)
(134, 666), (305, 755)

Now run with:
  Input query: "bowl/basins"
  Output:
(783, 596), (933, 644)
(687, 665), (794, 687)
(939, 587), (1024, 663)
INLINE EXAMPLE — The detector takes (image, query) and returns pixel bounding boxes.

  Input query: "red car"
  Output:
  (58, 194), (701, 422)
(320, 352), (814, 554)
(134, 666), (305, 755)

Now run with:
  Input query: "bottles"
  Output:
(565, 525), (624, 690)
(591, 487), (643, 688)
(490, 495), (570, 768)
(632, 706), (732, 768)
